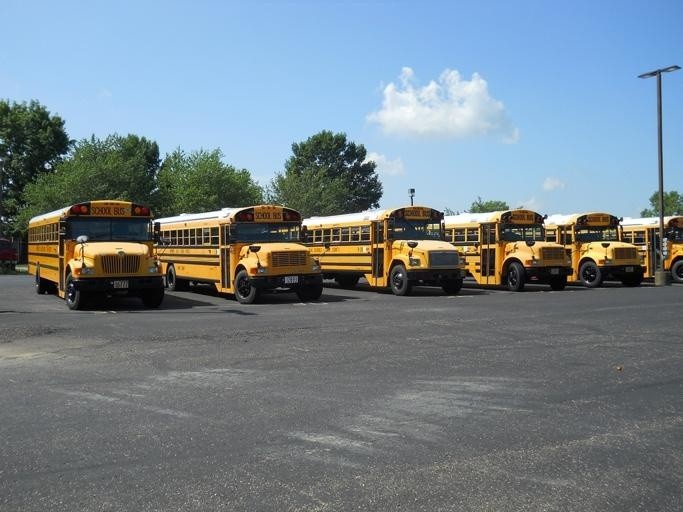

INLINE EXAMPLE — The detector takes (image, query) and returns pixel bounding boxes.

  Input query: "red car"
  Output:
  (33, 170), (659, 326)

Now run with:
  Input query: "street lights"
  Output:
(408, 188), (415, 206)
(638, 64), (681, 284)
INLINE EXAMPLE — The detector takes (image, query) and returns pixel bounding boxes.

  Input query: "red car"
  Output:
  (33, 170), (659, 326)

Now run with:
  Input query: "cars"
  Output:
(0, 239), (18, 270)
